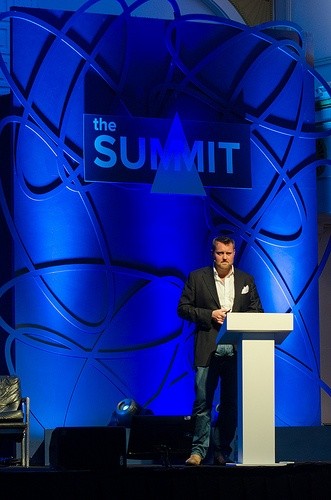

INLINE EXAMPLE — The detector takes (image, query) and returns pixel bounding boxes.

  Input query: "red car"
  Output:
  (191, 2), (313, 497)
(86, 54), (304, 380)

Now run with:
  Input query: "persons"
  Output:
(176, 235), (265, 465)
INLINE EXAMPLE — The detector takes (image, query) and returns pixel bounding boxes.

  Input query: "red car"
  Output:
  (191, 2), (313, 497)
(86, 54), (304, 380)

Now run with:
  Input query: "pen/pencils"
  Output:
(225, 308), (231, 314)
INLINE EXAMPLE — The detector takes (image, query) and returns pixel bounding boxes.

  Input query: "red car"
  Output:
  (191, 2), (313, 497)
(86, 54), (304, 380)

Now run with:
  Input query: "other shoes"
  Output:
(215, 455), (227, 465)
(185, 453), (201, 465)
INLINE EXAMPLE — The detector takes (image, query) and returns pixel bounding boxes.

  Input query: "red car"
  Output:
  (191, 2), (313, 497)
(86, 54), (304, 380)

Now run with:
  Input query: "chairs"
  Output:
(0, 374), (30, 469)
